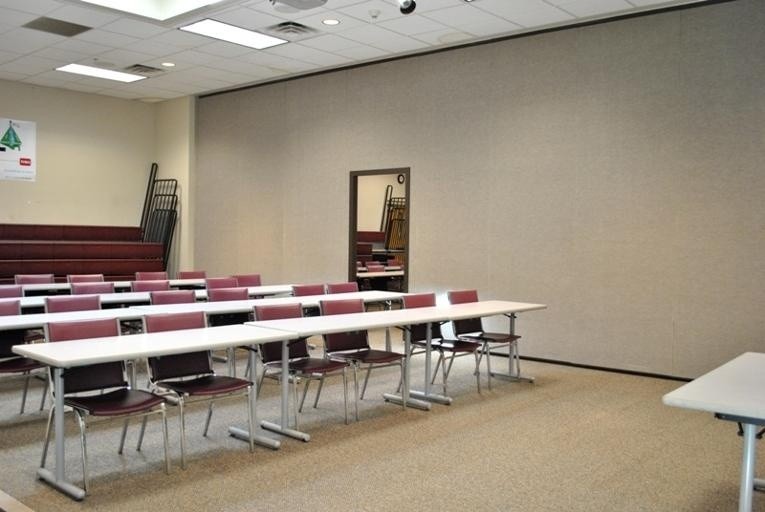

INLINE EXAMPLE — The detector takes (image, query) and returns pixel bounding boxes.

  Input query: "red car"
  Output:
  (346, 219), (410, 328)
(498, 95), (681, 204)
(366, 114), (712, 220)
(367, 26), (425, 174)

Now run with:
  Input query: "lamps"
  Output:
(393, 0), (418, 16)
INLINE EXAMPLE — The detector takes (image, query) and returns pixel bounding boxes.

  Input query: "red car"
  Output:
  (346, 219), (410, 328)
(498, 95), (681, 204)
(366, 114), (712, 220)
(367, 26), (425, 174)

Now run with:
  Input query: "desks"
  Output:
(660, 348), (765, 511)
